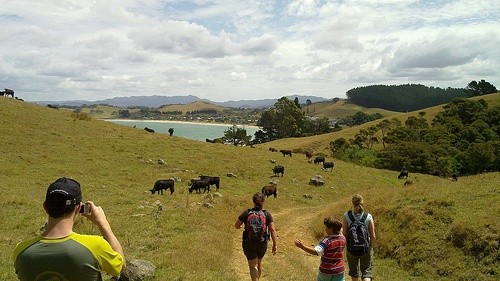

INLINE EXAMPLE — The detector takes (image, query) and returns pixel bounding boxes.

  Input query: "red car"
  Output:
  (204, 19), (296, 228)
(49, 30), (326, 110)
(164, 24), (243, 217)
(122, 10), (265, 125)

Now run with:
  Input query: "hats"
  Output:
(46, 176), (82, 206)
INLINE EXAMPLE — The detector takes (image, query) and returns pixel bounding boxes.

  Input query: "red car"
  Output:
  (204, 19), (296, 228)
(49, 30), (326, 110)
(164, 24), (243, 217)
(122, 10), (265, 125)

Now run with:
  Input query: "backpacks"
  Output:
(343, 210), (370, 257)
(245, 208), (267, 243)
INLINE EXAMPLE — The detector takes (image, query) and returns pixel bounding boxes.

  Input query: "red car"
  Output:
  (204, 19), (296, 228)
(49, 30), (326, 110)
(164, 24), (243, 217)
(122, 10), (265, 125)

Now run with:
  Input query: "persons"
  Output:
(13, 177), (126, 281)
(343, 195), (375, 281)
(235, 192), (277, 281)
(295, 217), (346, 281)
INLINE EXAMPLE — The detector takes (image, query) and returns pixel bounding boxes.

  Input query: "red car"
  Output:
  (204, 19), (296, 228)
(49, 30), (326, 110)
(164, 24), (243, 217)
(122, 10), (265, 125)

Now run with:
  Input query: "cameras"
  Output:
(78, 204), (89, 214)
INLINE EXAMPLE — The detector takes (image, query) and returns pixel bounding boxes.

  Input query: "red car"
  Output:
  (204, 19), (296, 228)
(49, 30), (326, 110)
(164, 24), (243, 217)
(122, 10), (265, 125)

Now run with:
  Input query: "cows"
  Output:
(261, 185), (279, 199)
(167, 126), (175, 137)
(272, 164), (285, 177)
(268, 146), (278, 153)
(451, 172), (458, 183)
(143, 126), (155, 134)
(323, 161), (335, 173)
(0, 88), (25, 102)
(148, 178), (175, 196)
(47, 103), (59, 110)
(249, 145), (256, 149)
(188, 173), (221, 195)
(279, 149), (292, 157)
(205, 137), (217, 144)
(313, 156), (325, 165)
(305, 149), (312, 160)
(396, 165), (413, 187)
